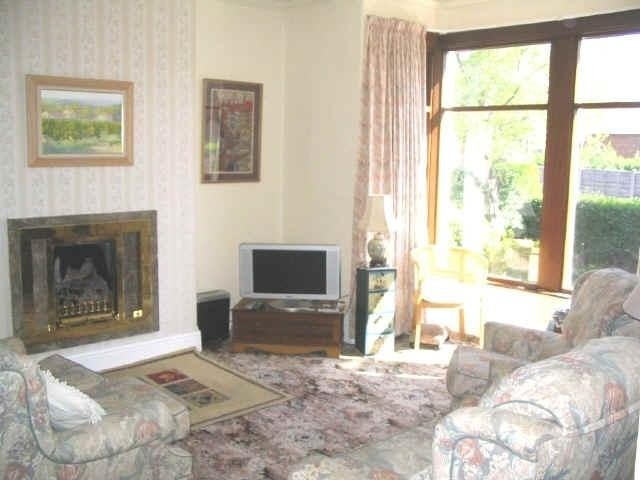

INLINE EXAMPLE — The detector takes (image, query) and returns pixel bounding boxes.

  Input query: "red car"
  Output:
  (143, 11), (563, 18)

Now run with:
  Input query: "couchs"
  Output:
(0, 335), (193, 480)
(287, 267), (640, 480)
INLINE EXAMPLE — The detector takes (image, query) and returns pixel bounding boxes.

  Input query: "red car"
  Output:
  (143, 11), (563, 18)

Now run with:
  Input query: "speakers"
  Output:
(197, 289), (231, 344)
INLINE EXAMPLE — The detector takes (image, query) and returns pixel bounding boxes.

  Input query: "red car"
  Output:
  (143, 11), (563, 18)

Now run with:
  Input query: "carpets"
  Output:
(97, 334), (460, 480)
(96, 345), (296, 430)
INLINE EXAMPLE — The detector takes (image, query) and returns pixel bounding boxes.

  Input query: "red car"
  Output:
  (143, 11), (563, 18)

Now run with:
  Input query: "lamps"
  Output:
(355, 195), (399, 268)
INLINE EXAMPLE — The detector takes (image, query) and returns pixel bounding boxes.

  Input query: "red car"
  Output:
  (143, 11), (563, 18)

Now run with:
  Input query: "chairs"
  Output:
(409, 244), (490, 348)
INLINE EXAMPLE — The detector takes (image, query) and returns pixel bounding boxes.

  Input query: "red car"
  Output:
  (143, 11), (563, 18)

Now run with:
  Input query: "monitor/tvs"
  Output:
(238, 243), (342, 301)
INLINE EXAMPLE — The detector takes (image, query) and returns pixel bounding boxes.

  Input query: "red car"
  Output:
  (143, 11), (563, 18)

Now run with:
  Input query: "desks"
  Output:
(229, 298), (345, 359)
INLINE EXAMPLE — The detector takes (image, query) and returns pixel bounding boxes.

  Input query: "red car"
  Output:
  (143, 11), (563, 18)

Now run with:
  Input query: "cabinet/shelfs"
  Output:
(357, 267), (397, 356)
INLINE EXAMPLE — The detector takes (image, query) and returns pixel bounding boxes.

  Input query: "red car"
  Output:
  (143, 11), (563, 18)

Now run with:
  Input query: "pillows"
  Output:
(43, 368), (108, 433)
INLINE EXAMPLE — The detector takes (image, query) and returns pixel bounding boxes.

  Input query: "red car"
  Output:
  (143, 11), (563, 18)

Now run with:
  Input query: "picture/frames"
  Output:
(25, 74), (135, 168)
(198, 78), (264, 185)
